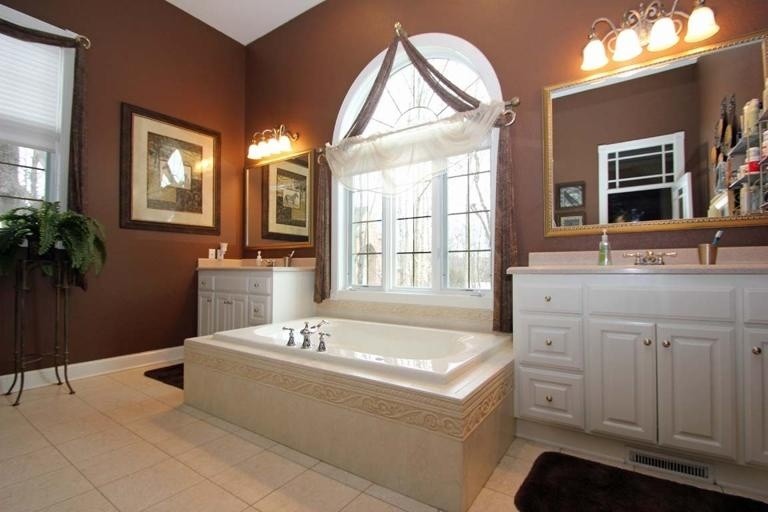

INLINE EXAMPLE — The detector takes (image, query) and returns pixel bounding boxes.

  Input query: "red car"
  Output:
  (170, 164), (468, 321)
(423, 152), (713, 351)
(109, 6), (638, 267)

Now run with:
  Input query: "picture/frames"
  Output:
(260, 159), (308, 243)
(555, 181), (587, 228)
(120, 101), (221, 236)
(241, 148), (317, 251)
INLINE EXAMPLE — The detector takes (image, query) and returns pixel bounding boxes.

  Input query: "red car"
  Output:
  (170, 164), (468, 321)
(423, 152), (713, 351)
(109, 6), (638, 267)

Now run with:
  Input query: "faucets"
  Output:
(261, 259), (273, 267)
(299, 318), (330, 349)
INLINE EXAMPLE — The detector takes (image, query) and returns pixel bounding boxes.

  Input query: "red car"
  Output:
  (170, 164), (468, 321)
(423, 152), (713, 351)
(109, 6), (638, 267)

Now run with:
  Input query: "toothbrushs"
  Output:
(712, 231), (724, 246)
(290, 250), (295, 258)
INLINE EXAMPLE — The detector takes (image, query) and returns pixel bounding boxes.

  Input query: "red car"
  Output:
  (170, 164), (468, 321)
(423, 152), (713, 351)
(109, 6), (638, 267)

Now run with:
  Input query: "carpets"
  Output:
(515, 449), (768, 510)
(145, 362), (183, 390)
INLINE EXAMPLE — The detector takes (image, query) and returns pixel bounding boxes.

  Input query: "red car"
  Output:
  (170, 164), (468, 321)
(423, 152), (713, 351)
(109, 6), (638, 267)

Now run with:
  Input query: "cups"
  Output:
(697, 243), (718, 264)
(283, 256), (291, 267)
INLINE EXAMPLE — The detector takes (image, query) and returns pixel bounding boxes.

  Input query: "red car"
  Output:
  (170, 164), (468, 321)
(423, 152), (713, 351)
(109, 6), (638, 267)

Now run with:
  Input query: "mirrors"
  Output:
(544, 28), (767, 238)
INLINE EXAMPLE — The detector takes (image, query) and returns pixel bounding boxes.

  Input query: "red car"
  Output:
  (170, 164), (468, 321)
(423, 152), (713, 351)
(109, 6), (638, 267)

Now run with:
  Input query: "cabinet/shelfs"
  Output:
(658, 283), (735, 461)
(198, 277), (313, 339)
(727, 109), (767, 217)
(736, 284), (768, 469)
(583, 284), (659, 448)
(513, 285), (582, 432)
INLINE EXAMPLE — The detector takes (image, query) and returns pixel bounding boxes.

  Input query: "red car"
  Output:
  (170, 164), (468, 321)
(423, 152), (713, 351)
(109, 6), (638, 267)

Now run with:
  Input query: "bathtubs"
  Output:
(213, 316), (512, 381)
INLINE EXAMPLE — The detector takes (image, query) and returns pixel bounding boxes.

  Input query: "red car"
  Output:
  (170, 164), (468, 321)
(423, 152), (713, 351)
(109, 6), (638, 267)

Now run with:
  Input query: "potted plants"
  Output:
(0, 200), (107, 289)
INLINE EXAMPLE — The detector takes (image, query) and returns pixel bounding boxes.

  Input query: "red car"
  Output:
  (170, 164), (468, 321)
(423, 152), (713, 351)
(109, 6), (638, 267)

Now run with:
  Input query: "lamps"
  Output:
(580, 2), (721, 71)
(248, 122), (299, 160)
